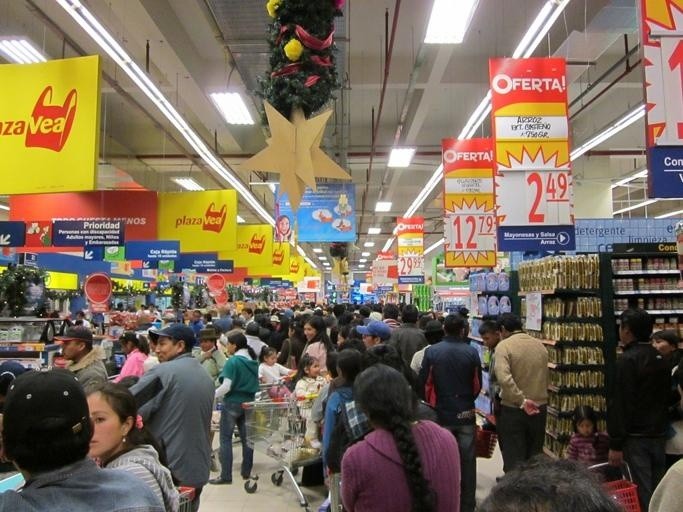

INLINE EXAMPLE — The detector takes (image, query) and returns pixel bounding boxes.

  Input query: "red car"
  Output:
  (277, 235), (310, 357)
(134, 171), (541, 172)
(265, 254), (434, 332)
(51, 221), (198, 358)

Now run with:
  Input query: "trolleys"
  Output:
(210, 372), (323, 511)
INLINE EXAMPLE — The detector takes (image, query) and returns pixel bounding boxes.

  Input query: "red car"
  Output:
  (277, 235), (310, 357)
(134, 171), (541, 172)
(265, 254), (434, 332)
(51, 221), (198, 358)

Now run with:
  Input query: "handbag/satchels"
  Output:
(283, 353), (296, 370)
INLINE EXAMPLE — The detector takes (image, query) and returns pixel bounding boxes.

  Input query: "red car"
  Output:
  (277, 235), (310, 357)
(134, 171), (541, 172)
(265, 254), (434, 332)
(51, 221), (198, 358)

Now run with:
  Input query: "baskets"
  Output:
(600, 480), (641, 512)
(474, 426), (499, 458)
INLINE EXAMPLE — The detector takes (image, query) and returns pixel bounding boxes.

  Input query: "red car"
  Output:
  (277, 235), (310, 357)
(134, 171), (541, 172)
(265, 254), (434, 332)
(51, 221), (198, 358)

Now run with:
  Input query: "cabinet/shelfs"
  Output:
(429, 251), (683, 460)
(1, 316), (68, 371)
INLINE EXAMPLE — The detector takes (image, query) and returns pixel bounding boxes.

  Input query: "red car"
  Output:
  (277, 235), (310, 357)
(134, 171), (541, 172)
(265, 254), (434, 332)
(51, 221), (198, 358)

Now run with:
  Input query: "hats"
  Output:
(1, 361), (90, 440)
(270, 315), (281, 323)
(196, 328), (222, 341)
(356, 320), (392, 340)
(148, 322), (197, 345)
(53, 326), (93, 342)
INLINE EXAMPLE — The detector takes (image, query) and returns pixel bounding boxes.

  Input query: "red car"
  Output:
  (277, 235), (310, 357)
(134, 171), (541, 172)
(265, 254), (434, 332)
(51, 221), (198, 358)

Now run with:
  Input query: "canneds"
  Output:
(611, 258), (683, 310)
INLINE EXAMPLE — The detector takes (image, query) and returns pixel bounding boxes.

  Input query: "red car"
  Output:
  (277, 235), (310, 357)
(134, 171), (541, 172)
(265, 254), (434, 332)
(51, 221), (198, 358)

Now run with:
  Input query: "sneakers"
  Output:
(208, 476), (232, 484)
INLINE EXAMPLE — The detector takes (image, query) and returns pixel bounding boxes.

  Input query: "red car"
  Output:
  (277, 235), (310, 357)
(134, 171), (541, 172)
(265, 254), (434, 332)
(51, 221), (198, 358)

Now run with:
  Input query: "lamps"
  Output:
(423, 237), (446, 258)
(153, 88), (204, 173)
(57, 0), (155, 106)
(456, 84), (491, 140)
(385, 148), (415, 170)
(382, 226), (400, 252)
(613, 198), (683, 217)
(421, 1), (478, 44)
(313, 248), (323, 254)
(364, 243), (376, 248)
(507, 47), (539, 58)
(368, 228), (381, 235)
(610, 166), (647, 191)
(374, 201), (392, 213)
(169, 176), (204, 193)
(208, 87), (257, 127)
(318, 256), (332, 270)
(1, 35), (49, 64)
(569, 98), (645, 167)
(400, 157), (442, 217)
(262, 207), (318, 269)
(236, 214), (246, 224)
(358, 252), (370, 269)
(204, 142), (262, 214)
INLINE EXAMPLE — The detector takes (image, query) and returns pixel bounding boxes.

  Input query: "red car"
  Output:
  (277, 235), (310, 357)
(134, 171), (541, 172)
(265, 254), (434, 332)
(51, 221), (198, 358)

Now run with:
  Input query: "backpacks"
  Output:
(325, 386), (375, 474)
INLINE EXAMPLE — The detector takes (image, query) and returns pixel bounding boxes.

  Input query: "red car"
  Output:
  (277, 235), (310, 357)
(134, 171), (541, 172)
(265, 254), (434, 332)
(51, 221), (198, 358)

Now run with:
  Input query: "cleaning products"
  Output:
(468, 268), (511, 413)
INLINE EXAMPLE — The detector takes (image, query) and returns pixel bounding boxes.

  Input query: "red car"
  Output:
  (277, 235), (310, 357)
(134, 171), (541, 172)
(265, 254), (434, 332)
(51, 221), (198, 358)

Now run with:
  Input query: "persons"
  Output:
(275, 215), (292, 241)
(568, 308), (683, 512)
(478, 454), (623, 511)
(0, 301), (357, 512)
(340, 301), (550, 511)
